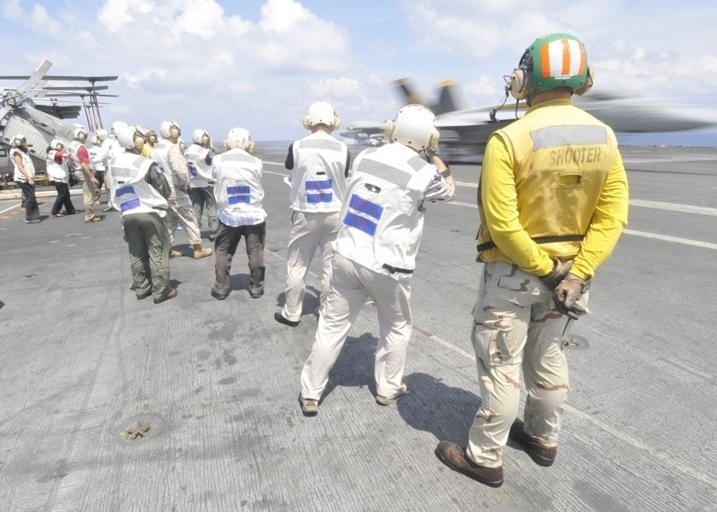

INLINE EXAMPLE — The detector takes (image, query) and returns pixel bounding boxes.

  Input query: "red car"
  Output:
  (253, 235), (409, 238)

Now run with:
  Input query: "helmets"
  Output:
(50, 139), (64, 150)
(504, 32), (593, 99)
(223, 127), (255, 153)
(383, 103), (440, 152)
(303, 101), (341, 131)
(10, 134), (26, 148)
(72, 127), (108, 145)
(111, 120), (213, 152)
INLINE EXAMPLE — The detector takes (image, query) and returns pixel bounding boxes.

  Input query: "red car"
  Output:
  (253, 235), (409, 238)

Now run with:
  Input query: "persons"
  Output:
(273, 102), (350, 326)
(436, 35), (632, 489)
(8, 134), (44, 223)
(108, 124), (177, 303)
(299, 102), (456, 415)
(45, 139), (81, 217)
(69, 121), (213, 261)
(211, 127), (269, 300)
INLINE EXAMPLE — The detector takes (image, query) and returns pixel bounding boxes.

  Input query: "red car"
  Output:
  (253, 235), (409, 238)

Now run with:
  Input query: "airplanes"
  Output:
(0, 57), (120, 183)
(340, 65), (717, 156)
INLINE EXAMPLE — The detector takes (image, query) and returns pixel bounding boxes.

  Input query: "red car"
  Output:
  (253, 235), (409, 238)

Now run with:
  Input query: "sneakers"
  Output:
(25, 218), (41, 224)
(102, 207), (115, 212)
(55, 212), (63, 217)
(211, 288), (227, 300)
(275, 312), (300, 327)
(194, 248), (213, 259)
(170, 250), (184, 258)
(299, 393), (318, 413)
(137, 290), (152, 299)
(73, 210), (81, 214)
(436, 440), (504, 488)
(154, 287), (176, 303)
(508, 419), (557, 466)
(377, 382), (408, 405)
(85, 216), (102, 223)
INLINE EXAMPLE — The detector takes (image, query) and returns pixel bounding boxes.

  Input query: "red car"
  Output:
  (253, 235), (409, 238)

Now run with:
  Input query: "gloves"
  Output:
(540, 256), (591, 321)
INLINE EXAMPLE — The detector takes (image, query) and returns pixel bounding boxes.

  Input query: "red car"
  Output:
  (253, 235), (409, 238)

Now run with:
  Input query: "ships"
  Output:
(0, 136), (716, 512)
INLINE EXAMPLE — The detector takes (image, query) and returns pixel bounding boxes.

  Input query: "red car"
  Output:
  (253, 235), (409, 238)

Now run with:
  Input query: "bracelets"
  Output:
(440, 166), (454, 176)
